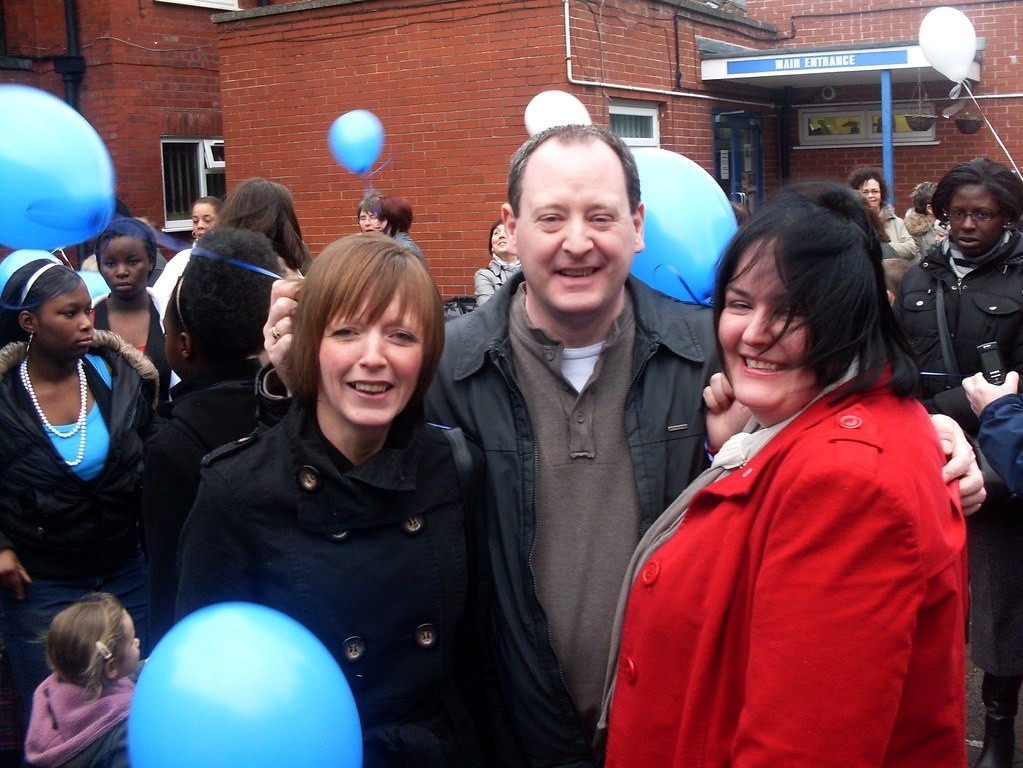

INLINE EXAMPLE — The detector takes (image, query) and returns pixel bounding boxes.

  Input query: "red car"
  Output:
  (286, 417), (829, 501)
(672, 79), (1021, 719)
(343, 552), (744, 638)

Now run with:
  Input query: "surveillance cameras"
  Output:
(821, 86), (836, 100)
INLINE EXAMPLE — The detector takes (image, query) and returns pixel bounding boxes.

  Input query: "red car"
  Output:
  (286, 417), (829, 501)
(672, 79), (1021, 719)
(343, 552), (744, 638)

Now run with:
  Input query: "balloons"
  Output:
(127, 602), (363, 768)
(328, 109), (385, 172)
(0, 83), (114, 252)
(919, 7), (976, 84)
(524, 90), (592, 135)
(628, 147), (739, 306)
(0, 248), (58, 295)
(76, 270), (111, 299)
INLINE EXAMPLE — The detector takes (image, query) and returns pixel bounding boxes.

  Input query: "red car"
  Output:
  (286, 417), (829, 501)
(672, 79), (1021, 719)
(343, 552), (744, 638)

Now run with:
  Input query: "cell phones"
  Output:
(977, 342), (1006, 386)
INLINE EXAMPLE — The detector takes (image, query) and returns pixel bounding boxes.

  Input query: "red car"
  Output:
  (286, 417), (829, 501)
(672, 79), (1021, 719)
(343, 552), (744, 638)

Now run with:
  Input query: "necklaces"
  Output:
(20, 356), (88, 467)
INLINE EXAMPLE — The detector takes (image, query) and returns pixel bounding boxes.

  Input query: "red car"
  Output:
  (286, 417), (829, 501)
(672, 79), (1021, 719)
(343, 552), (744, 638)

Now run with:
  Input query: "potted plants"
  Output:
(952, 111), (985, 133)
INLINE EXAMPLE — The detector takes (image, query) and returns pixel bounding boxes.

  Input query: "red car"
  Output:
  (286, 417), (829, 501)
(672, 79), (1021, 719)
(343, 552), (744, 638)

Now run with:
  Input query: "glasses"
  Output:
(944, 208), (1004, 223)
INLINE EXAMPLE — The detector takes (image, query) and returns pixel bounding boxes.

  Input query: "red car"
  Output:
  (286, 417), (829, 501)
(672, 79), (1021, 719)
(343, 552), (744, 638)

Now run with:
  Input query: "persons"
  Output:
(594, 178), (969, 768)
(0, 262), (167, 728)
(26, 592), (149, 768)
(263, 123), (985, 768)
(848, 159), (1023, 768)
(133, 234), (286, 648)
(357, 191), (422, 258)
(474, 218), (522, 307)
(82, 178), (315, 361)
(175, 232), (526, 768)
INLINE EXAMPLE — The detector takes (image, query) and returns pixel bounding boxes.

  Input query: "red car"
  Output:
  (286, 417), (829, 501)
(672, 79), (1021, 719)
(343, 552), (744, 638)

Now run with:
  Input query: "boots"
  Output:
(974, 698), (1018, 768)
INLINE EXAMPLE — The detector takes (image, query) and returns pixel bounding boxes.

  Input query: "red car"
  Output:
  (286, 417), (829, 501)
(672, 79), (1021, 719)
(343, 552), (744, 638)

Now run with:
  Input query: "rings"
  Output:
(272, 327), (280, 341)
